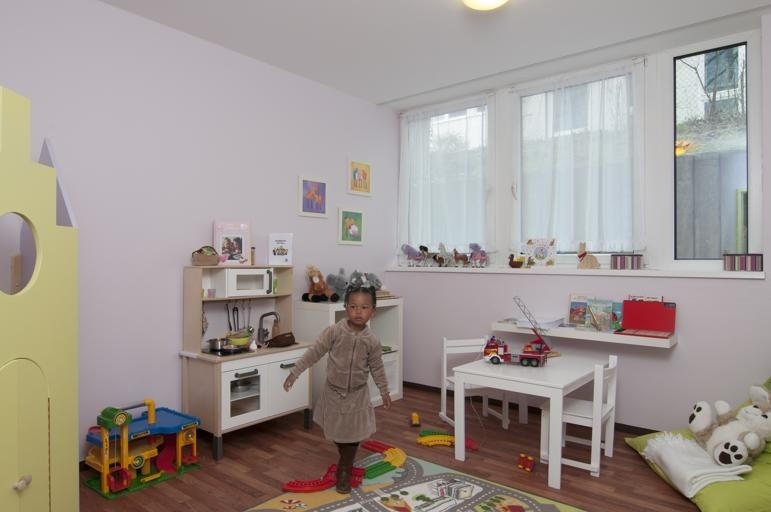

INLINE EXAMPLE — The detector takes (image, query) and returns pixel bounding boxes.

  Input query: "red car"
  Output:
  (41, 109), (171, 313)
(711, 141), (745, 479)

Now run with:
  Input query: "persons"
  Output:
(284, 285), (392, 494)
(222, 237), (242, 254)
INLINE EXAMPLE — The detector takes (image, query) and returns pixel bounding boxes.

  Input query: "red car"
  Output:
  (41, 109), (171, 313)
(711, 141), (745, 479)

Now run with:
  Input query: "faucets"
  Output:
(256, 311), (281, 345)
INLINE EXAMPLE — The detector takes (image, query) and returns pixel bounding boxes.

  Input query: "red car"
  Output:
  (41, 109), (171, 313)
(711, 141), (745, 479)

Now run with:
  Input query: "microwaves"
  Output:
(210, 267), (274, 299)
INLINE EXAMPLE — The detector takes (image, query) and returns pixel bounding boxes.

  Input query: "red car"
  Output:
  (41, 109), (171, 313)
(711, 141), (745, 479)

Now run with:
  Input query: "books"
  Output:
(569, 294), (623, 330)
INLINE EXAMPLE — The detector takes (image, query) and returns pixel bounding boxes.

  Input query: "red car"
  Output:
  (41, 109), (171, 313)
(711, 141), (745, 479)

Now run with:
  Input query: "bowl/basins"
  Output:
(264, 332), (295, 348)
(228, 336), (249, 345)
(231, 380), (250, 392)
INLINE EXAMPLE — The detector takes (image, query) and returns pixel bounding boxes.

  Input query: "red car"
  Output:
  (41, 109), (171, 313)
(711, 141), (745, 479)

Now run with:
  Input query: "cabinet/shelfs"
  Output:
(182, 341), (312, 460)
(183, 262), (293, 350)
(298, 295), (403, 431)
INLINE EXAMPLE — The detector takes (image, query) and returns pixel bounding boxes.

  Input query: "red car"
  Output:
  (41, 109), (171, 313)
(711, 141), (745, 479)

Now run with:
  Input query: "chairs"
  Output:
(540, 354), (619, 478)
(440, 334), (511, 433)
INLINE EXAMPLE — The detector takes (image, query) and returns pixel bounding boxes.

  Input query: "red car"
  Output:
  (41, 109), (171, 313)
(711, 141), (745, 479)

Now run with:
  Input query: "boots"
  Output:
(336, 448), (357, 493)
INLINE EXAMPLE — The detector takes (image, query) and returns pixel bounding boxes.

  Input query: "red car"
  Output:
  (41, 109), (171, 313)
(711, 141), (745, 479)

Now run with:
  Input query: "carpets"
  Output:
(240, 449), (587, 512)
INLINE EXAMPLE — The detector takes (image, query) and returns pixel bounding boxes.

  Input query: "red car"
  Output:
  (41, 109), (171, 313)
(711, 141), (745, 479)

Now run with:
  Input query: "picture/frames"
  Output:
(338, 207), (364, 246)
(525, 238), (558, 268)
(299, 176), (328, 218)
(347, 161), (373, 197)
(211, 220), (252, 266)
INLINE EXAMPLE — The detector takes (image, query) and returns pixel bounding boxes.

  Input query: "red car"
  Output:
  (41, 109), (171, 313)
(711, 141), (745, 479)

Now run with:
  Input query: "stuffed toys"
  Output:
(577, 242), (601, 269)
(303, 266), (383, 302)
(689, 385), (771, 466)
(402, 242), (490, 268)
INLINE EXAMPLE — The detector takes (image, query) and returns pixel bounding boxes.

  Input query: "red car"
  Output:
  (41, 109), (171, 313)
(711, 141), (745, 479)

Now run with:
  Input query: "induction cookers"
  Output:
(201, 348), (241, 357)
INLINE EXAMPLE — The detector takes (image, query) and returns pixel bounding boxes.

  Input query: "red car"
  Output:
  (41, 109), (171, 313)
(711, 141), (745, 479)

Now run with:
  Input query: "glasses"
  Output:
(344, 303), (375, 310)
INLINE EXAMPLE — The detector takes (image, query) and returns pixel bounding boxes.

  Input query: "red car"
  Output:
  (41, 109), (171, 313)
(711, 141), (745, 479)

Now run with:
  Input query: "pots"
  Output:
(205, 338), (231, 351)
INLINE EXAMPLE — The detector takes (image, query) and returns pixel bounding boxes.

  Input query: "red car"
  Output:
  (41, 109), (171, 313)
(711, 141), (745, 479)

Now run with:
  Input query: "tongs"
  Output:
(232, 307), (239, 332)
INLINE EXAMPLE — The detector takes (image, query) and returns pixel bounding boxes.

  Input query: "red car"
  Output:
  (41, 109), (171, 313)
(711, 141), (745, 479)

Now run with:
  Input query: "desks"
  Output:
(453, 352), (612, 490)
